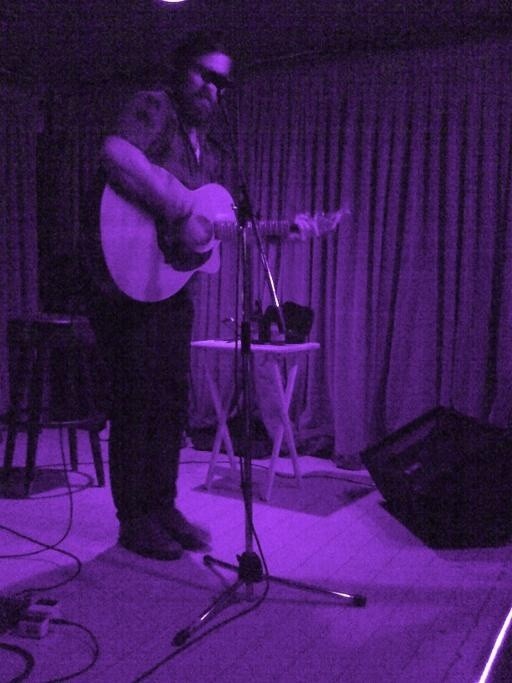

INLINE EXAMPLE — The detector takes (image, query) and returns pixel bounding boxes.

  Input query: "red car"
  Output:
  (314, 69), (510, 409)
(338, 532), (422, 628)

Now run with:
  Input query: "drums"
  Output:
(5, 314), (119, 447)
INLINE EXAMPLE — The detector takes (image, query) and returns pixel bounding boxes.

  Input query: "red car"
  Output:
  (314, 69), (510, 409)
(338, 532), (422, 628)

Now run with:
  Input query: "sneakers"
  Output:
(160, 504), (210, 553)
(115, 508), (185, 562)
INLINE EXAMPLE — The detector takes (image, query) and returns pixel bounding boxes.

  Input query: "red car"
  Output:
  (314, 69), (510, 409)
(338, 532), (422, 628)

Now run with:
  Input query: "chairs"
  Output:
(3, 320), (107, 494)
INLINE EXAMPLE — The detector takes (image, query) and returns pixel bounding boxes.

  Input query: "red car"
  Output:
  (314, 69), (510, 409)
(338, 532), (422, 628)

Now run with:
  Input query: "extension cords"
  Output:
(18, 594), (60, 638)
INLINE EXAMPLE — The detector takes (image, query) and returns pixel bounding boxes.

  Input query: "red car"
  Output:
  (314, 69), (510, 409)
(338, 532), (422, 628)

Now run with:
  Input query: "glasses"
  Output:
(190, 58), (237, 91)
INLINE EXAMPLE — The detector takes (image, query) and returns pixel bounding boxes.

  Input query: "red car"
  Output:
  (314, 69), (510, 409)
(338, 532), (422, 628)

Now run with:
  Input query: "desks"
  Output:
(190, 340), (322, 502)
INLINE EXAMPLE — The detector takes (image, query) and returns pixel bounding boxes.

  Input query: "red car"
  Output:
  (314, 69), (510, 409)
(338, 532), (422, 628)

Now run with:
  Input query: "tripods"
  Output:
(174, 91), (367, 644)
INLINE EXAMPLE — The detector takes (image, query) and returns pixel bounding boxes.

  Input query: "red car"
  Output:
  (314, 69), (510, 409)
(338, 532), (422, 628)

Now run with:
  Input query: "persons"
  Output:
(77, 28), (319, 560)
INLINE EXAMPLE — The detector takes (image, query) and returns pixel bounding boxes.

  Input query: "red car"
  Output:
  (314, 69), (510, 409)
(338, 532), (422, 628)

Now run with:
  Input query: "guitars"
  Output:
(87, 163), (351, 309)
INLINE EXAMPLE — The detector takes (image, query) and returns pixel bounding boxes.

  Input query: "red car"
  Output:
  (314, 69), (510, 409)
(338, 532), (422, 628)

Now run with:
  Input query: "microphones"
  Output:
(200, 67), (234, 91)
